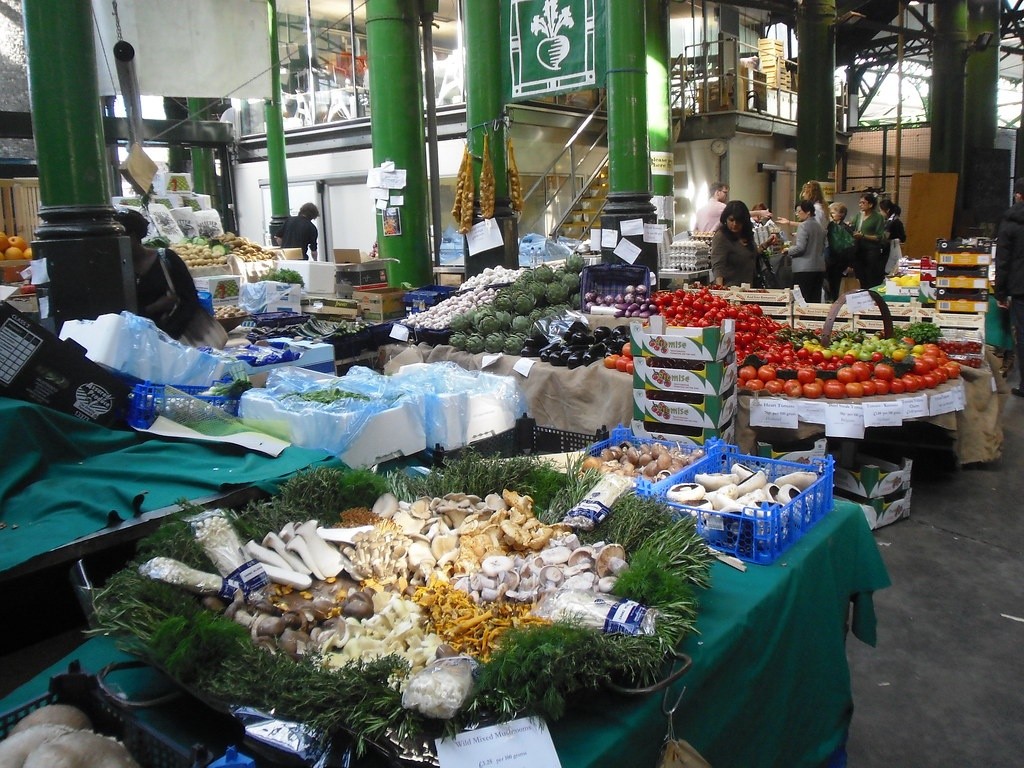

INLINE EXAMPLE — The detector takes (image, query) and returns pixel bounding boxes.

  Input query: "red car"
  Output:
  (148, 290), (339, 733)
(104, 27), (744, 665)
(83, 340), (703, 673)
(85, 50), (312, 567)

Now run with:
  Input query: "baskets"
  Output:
(126, 375), (240, 431)
(650, 439), (836, 565)
(581, 262), (650, 313)
(0, 657), (213, 768)
(252, 311), (310, 328)
(588, 423), (718, 502)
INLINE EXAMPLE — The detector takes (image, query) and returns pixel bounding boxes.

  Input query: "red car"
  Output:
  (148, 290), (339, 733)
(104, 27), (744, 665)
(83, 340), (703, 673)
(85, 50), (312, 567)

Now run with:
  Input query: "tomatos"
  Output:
(602, 289), (961, 399)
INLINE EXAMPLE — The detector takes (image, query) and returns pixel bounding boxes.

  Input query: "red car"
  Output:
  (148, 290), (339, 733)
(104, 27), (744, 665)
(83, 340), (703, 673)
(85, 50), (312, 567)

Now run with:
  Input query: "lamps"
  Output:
(831, 12), (867, 34)
(968, 32), (994, 56)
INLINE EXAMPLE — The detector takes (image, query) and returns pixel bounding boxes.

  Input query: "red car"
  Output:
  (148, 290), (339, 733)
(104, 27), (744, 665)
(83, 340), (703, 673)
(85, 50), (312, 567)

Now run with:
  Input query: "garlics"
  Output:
(450, 164), (522, 233)
(399, 264), (555, 330)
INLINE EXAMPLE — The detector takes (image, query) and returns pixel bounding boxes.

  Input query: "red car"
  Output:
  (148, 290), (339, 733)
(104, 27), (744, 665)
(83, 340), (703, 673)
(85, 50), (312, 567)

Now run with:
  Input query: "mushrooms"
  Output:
(580, 441), (818, 546)
(0, 703), (146, 768)
(139, 488), (631, 700)
(212, 304), (247, 317)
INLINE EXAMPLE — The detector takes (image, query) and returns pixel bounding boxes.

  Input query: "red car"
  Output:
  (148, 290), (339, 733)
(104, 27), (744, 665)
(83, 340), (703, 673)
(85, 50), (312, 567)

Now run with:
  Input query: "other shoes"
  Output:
(998, 356), (1014, 373)
(1012, 386), (1024, 396)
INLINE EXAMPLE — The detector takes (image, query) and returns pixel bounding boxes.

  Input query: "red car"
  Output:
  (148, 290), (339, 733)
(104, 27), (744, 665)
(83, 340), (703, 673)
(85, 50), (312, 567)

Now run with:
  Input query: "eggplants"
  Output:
(584, 284), (658, 318)
(519, 323), (630, 369)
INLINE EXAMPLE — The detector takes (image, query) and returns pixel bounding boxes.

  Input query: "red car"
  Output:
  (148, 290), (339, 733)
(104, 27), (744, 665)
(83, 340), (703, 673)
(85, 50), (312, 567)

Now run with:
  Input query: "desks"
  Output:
(435, 57), (466, 106)
(382, 344), (969, 471)
(659, 269), (710, 284)
(1, 424), (893, 767)
(288, 85), (369, 128)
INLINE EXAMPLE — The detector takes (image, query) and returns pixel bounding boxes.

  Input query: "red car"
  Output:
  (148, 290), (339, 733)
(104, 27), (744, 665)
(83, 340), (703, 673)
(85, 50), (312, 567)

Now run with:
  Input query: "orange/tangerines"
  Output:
(0, 231), (32, 284)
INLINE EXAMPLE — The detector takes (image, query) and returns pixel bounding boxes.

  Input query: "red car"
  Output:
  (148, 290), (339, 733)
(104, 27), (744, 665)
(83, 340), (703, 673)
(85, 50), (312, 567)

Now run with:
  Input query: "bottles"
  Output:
(529, 247), (536, 269)
(536, 248), (543, 268)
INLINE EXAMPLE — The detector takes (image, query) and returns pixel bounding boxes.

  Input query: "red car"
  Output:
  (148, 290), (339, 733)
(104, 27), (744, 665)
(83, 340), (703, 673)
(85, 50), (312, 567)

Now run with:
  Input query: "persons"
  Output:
(994, 178), (1024, 397)
(774, 181), (906, 302)
(694, 182), (773, 233)
(712, 200), (776, 289)
(276, 202), (318, 261)
(117, 209), (198, 341)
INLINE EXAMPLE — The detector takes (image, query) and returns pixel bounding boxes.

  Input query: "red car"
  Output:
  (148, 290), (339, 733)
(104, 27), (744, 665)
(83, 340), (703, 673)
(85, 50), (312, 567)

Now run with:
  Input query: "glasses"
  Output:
(807, 181), (814, 188)
(720, 190), (727, 194)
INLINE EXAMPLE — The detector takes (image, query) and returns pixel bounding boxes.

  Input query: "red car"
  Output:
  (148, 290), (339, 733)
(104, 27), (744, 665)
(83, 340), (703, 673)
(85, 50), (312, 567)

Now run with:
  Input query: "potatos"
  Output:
(173, 233), (276, 267)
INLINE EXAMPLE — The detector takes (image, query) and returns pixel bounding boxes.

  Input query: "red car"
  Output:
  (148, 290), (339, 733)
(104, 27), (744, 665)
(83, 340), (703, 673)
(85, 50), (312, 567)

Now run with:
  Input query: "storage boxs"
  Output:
(0, 258), (39, 322)
(112, 367), (242, 429)
(755, 424), (956, 529)
(239, 374), (427, 470)
(431, 413), (609, 468)
(757, 37), (791, 89)
(647, 439), (836, 568)
(1, 660), (214, 768)
(699, 81), (730, 113)
(581, 262), (649, 312)
(629, 316), (738, 460)
(0, 301), (133, 430)
(686, 240), (994, 362)
(382, 362), (518, 452)
(582, 421), (724, 501)
(113, 172), (513, 377)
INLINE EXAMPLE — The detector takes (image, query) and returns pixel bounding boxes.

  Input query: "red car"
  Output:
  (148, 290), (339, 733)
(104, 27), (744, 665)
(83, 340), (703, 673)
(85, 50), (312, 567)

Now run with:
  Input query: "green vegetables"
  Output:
(282, 388), (368, 405)
(261, 268), (305, 288)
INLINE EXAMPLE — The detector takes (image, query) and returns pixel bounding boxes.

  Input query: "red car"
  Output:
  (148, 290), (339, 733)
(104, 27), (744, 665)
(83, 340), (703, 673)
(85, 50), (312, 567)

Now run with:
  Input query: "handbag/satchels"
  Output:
(858, 239), (882, 265)
(158, 247), (228, 350)
(775, 250), (793, 289)
(757, 252), (774, 288)
(827, 221), (856, 253)
(885, 239), (902, 277)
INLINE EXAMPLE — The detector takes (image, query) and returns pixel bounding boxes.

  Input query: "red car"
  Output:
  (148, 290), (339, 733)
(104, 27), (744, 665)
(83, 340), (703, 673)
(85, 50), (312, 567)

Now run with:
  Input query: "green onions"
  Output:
(197, 378), (254, 399)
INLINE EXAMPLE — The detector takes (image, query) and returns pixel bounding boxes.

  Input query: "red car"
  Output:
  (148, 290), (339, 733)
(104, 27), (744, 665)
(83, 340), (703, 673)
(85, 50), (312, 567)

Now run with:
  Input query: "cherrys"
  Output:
(932, 339), (983, 368)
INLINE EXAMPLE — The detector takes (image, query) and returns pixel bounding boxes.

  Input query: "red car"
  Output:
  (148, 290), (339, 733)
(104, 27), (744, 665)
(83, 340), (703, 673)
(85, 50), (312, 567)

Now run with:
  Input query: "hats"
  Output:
(1015, 177), (1024, 193)
